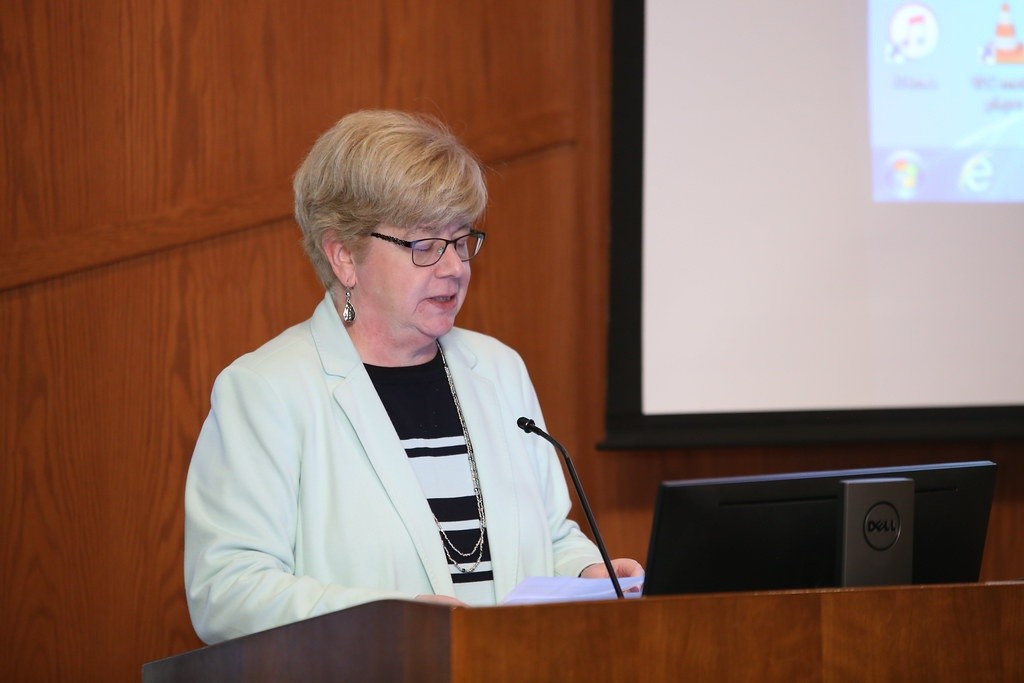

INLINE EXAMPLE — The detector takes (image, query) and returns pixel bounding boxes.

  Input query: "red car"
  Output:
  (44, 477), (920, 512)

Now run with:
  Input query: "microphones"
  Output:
(517, 416), (622, 596)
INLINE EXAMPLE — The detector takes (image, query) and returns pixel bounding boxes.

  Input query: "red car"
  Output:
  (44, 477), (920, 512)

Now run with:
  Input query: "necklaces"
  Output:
(425, 338), (486, 574)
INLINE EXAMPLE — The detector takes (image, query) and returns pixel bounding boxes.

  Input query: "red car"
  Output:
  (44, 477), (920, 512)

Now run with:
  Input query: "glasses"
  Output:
(367, 228), (486, 267)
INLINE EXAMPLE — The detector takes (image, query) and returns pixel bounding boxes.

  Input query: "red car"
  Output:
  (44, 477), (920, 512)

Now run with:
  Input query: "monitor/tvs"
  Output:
(643, 462), (998, 594)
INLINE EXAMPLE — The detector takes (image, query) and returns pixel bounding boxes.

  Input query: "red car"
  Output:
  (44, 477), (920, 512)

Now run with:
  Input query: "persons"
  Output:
(181, 106), (646, 646)
